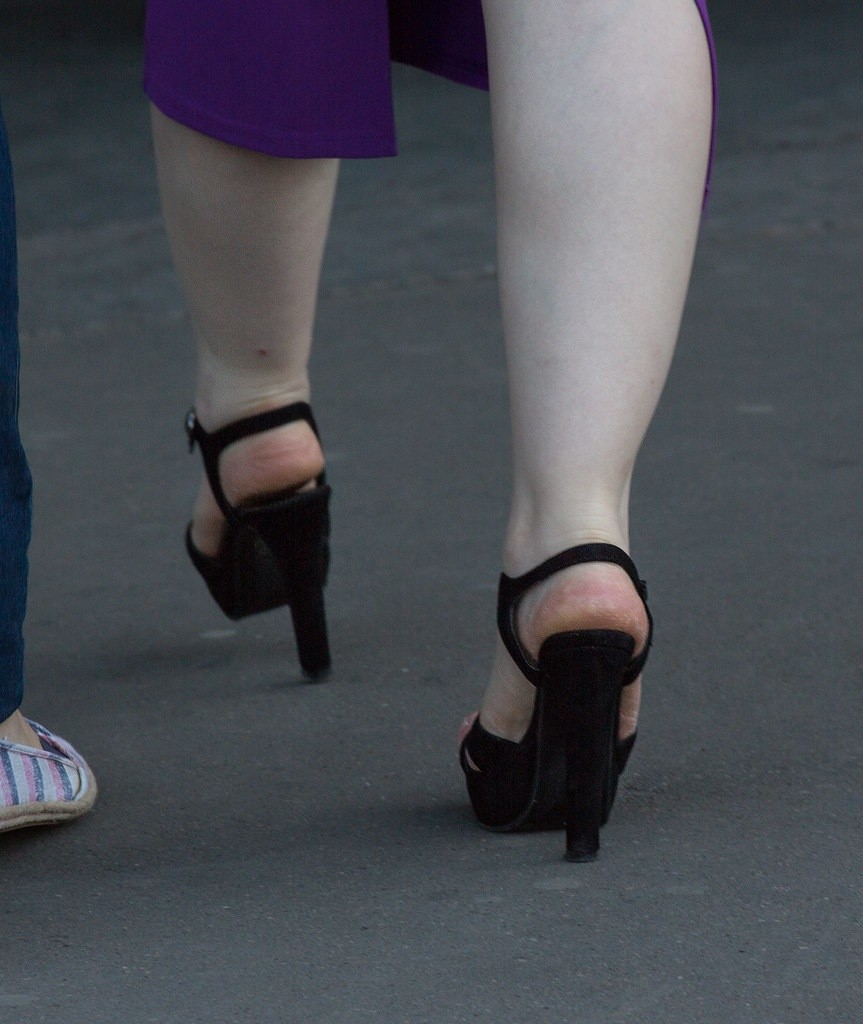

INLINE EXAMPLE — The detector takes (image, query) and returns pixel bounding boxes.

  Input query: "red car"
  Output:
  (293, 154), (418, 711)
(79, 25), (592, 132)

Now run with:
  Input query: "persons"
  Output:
(122, 0), (719, 864)
(0, 122), (99, 838)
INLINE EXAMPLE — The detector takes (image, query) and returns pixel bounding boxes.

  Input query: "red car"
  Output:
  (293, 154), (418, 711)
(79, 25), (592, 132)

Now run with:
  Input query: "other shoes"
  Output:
(0, 716), (99, 833)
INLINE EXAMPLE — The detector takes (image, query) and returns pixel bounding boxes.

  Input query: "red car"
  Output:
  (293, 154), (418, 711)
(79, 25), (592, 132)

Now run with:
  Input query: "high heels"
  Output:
(460, 542), (654, 861)
(184, 401), (332, 683)
